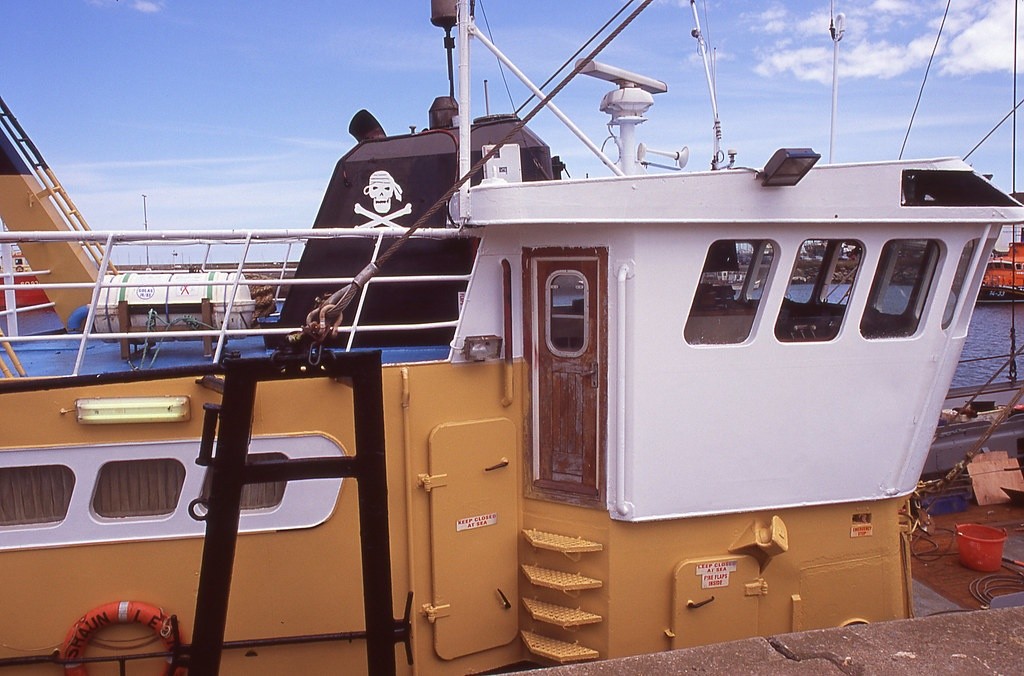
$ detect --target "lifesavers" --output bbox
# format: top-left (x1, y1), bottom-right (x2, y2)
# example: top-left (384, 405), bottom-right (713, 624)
top-left (62, 600), bottom-right (185, 676)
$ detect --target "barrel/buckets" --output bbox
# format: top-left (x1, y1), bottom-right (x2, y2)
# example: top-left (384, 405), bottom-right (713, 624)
top-left (954, 524), bottom-right (1007, 572)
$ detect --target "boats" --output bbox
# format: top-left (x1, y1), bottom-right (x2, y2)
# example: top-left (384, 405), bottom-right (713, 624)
top-left (0, 244), bottom-right (67, 337)
top-left (1, 0), bottom-right (1024, 675)
top-left (951, 163), bottom-right (1024, 307)
top-left (804, 240), bottom-right (851, 258)
